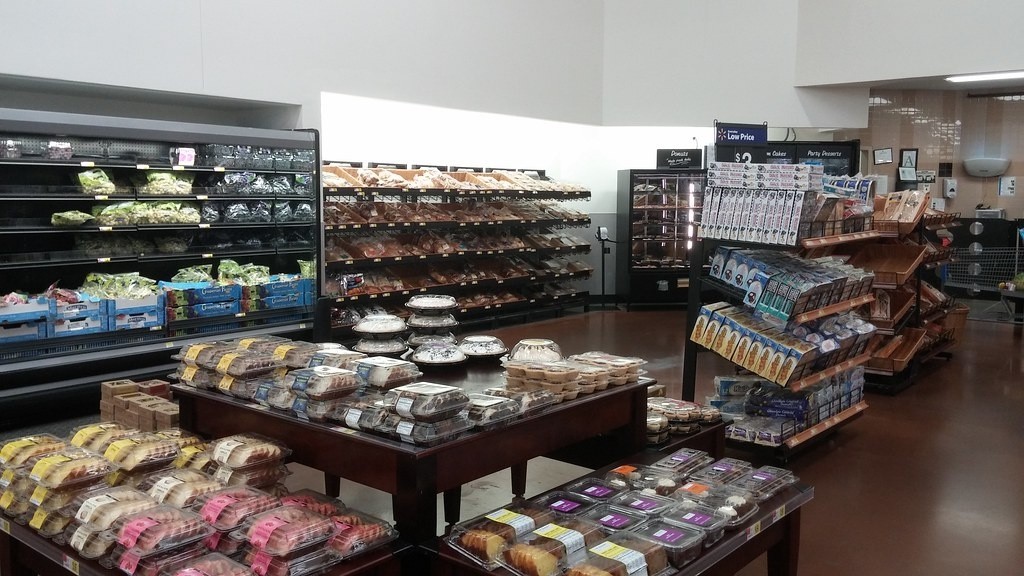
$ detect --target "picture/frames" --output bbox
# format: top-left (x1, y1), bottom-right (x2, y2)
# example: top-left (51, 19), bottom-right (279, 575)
top-left (873, 147), bottom-right (894, 165)
top-left (898, 166), bottom-right (918, 182)
top-left (899, 148), bottom-right (919, 171)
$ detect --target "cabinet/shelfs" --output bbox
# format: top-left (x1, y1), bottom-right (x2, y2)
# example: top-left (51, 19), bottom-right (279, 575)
top-left (0, 107), bottom-right (321, 436)
top-left (615, 168), bottom-right (728, 311)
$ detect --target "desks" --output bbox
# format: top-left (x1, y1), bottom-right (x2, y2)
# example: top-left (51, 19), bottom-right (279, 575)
top-left (420, 446), bottom-right (816, 576)
top-left (0, 509), bottom-right (413, 576)
top-left (646, 396), bottom-right (734, 462)
top-left (171, 352), bottom-right (657, 576)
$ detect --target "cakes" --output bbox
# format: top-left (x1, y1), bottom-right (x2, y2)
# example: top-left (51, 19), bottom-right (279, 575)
top-left (354, 295), bottom-right (561, 365)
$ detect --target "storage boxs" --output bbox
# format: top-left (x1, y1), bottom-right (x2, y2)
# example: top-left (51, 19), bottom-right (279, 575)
top-left (322, 165), bottom-right (592, 342)
top-left (689, 159), bottom-right (948, 447)
top-left (0, 273), bottom-right (317, 364)
top-left (101, 379), bottom-right (184, 433)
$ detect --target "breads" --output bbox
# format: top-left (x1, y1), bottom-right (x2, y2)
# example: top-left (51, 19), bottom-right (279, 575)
top-left (0, 421), bottom-right (389, 576)
top-left (457, 504), bottom-right (668, 576)
top-left (175, 335), bottom-right (554, 445)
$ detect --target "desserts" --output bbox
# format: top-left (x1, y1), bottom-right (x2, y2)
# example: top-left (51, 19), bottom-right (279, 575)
top-left (644, 397), bottom-right (720, 442)
top-left (503, 353), bottom-right (642, 400)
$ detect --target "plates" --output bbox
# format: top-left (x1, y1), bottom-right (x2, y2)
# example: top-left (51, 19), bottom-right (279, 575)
top-left (351, 302), bottom-right (509, 369)
